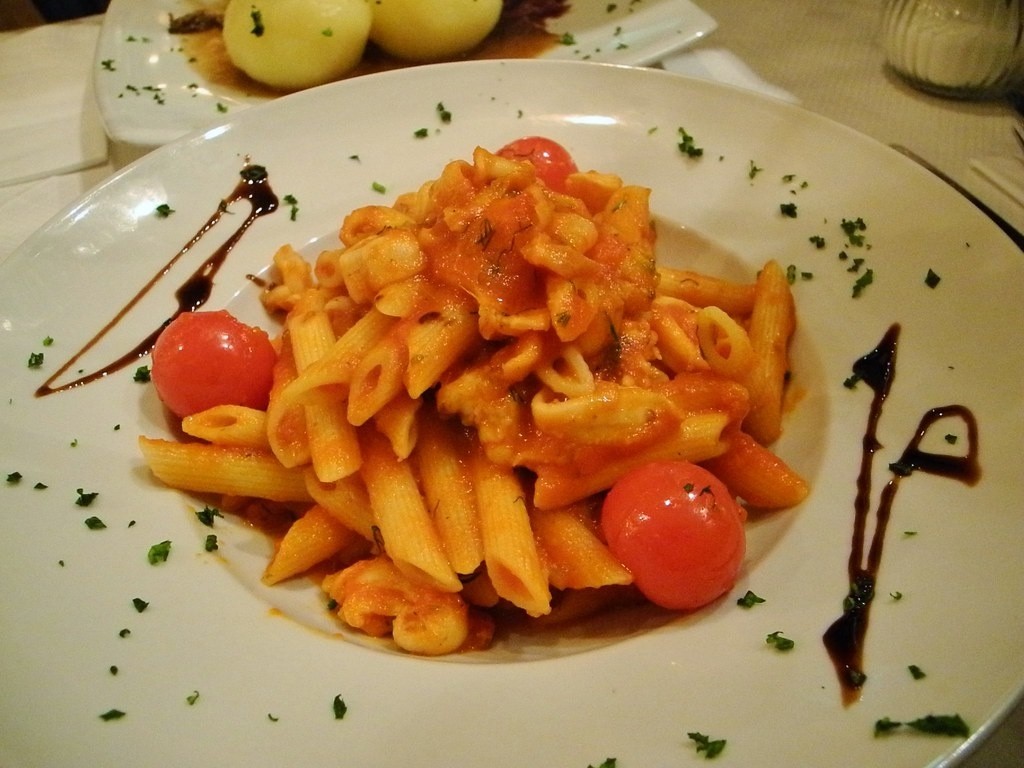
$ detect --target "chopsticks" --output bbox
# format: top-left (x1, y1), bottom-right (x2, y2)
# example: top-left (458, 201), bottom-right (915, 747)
top-left (968, 154), bottom-right (1024, 208)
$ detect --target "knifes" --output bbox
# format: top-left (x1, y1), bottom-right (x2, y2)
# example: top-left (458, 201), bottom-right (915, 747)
top-left (891, 144), bottom-right (1024, 251)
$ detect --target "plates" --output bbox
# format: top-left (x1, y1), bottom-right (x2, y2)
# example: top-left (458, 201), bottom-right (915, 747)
top-left (92, 0), bottom-right (719, 146)
top-left (0, 58), bottom-right (1024, 768)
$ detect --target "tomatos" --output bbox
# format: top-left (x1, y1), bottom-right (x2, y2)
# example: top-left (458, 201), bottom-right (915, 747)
top-left (147, 309), bottom-right (277, 418)
top-left (600, 460), bottom-right (745, 610)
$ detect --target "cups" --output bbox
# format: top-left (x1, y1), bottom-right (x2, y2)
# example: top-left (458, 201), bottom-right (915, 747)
top-left (879, 0), bottom-right (1024, 99)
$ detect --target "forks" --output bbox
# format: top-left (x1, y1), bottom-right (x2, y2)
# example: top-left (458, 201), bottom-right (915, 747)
top-left (1012, 119), bottom-right (1024, 153)
